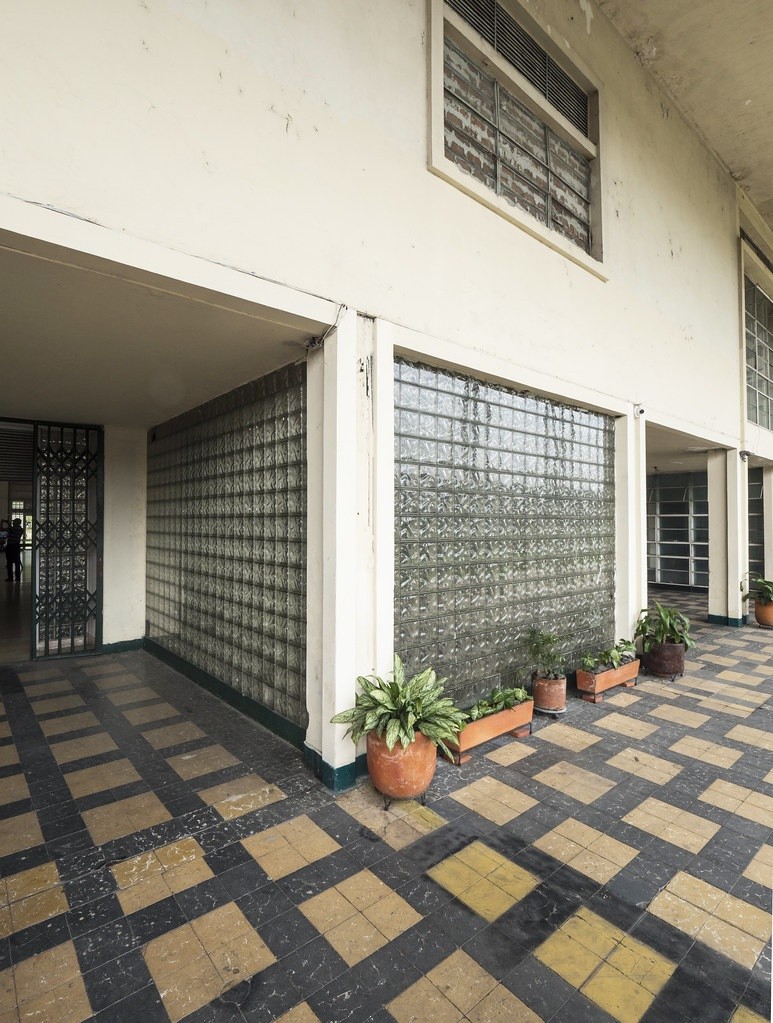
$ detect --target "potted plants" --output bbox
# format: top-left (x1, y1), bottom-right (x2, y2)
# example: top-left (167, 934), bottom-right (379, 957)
top-left (516, 624), bottom-right (574, 722)
top-left (634, 597), bottom-right (695, 683)
top-left (438, 686), bottom-right (537, 766)
top-left (739, 570), bottom-right (773, 628)
top-left (574, 638), bottom-right (639, 700)
top-left (330, 651), bottom-right (468, 812)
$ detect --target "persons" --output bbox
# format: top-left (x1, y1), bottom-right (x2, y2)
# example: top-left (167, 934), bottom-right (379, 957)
top-left (5, 518), bottom-right (23, 581)
